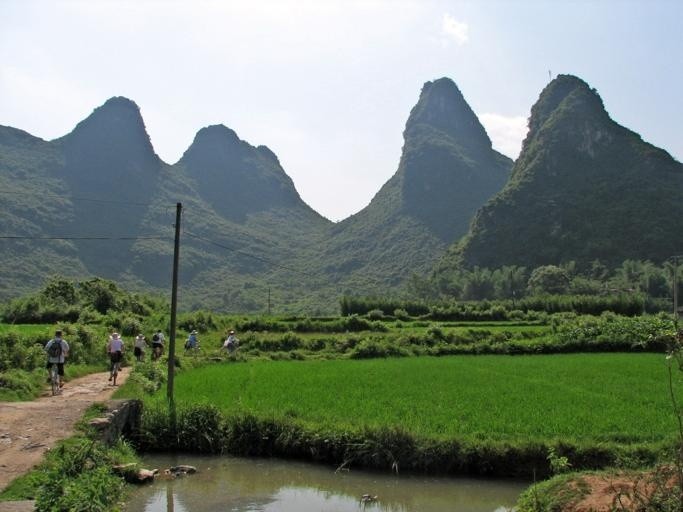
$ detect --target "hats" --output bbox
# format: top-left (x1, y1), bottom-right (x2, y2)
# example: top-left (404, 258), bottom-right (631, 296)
top-left (136, 334), bottom-right (144, 340)
top-left (190, 330), bottom-right (198, 335)
top-left (109, 333), bottom-right (121, 340)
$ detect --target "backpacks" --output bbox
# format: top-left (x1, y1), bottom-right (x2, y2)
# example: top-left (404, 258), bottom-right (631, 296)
top-left (47, 339), bottom-right (61, 361)
top-left (152, 334), bottom-right (159, 341)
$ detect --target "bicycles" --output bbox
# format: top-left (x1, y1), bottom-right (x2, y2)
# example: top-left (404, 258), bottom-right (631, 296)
top-left (135, 340), bottom-right (248, 363)
top-left (50, 364), bottom-right (63, 395)
top-left (112, 361), bottom-right (122, 385)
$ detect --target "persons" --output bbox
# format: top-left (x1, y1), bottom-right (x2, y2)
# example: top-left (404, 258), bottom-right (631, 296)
top-left (105, 332), bottom-right (127, 382)
top-left (222, 329), bottom-right (239, 354)
top-left (43, 329), bottom-right (70, 387)
top-left (132, 333), bottom-right (147, 361)
top-left (152, 328), bottom-right (167, 357)
top-left (183, 329), bottom-right (199, 352)
